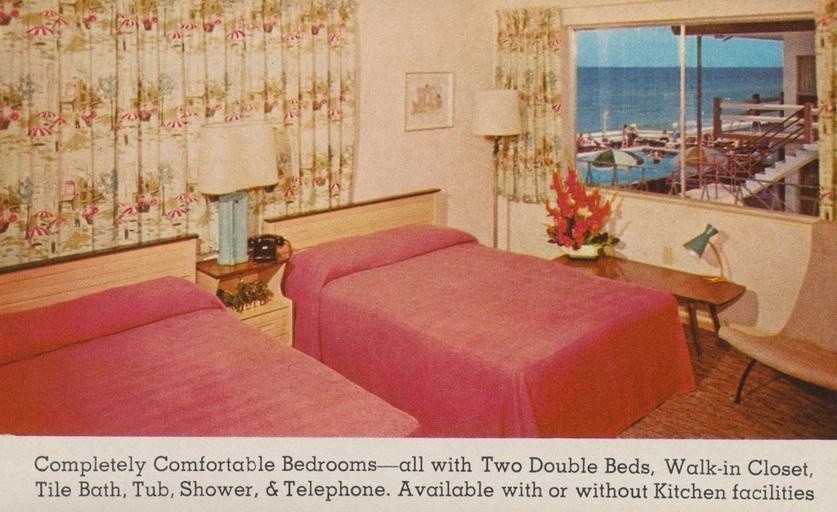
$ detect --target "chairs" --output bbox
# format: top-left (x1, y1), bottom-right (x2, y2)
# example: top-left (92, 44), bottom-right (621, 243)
top-left (718, 220), bottom-right (837, 405)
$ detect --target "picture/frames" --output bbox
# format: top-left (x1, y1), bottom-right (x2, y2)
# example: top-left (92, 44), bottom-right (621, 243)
top-left (405, 72), bottom-right (456, 132)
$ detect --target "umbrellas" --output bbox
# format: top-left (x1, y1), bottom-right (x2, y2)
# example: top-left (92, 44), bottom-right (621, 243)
top-left (591, 149), bottom-right (642, 182)
top-left (670, 145), bottom-right (724, 172)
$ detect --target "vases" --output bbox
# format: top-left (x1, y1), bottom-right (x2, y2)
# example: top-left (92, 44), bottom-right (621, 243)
top-left (562, 245), bottom-right (600, 259)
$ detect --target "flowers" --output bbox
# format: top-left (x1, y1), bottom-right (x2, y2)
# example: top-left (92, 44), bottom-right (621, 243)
top-left (546, 167), bottom-right (619, 258)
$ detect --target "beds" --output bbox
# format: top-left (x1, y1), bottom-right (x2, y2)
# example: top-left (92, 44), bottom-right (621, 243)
top-left (0, 233), bottom-right (422, 437)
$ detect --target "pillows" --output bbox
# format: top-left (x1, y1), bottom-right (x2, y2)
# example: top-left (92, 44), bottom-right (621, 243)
top-left (0, 276), bottom-right (226, 366)
top-left (289, 223), bottom-right (479, 301)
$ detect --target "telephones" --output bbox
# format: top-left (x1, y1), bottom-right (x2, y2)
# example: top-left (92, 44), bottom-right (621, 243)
top-left (247, 234), bottom-right (284, 263)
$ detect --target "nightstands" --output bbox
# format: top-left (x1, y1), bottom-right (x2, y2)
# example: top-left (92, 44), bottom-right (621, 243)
top-left (197, 250), bottom-right (294, 348)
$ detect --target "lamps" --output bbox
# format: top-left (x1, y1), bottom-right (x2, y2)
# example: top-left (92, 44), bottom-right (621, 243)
top-left (470, 90), bottom-right (522, 249)
top-left (197, 120), bottom-right (278, 267)
top-left (684, 224), bottom-right (728, 284)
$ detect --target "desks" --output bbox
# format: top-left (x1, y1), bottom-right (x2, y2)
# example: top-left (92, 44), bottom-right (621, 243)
top-left (552, 255), bottom-right (745, 357)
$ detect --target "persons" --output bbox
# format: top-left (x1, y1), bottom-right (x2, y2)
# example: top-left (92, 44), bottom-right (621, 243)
top-left (629, 122), bottom-right (639, 146)
top-left (619, 124), bottom-right (633, 149)
top-left (647, 148), bottom-right (655, 158)
top-left (650, 150), bottom-right (661, 165)
top-left (587, 132), bottom-right (593, 140)
top-left (577, 133), bottom-right (584, 144)
